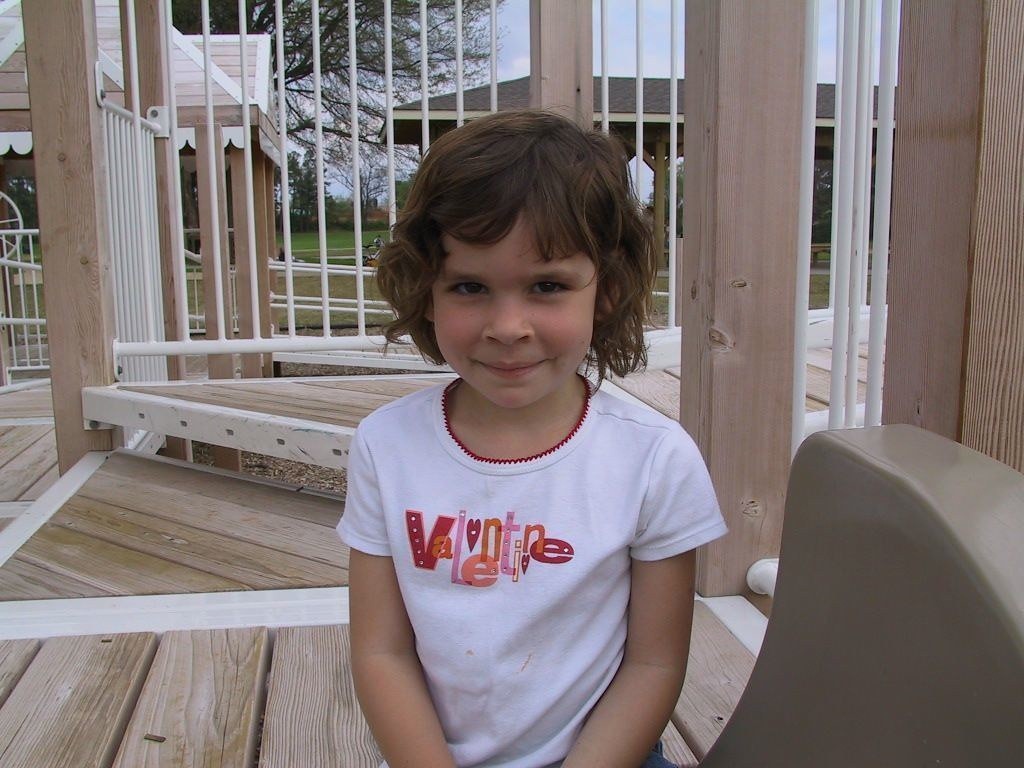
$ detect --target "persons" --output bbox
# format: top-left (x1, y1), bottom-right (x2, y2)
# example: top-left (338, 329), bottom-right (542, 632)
top-left (332, 108), bottom-right (728, 768)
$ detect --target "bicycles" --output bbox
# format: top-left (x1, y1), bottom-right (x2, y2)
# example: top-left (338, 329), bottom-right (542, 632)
top-left (344, 234), bottom-right (387, 268)
